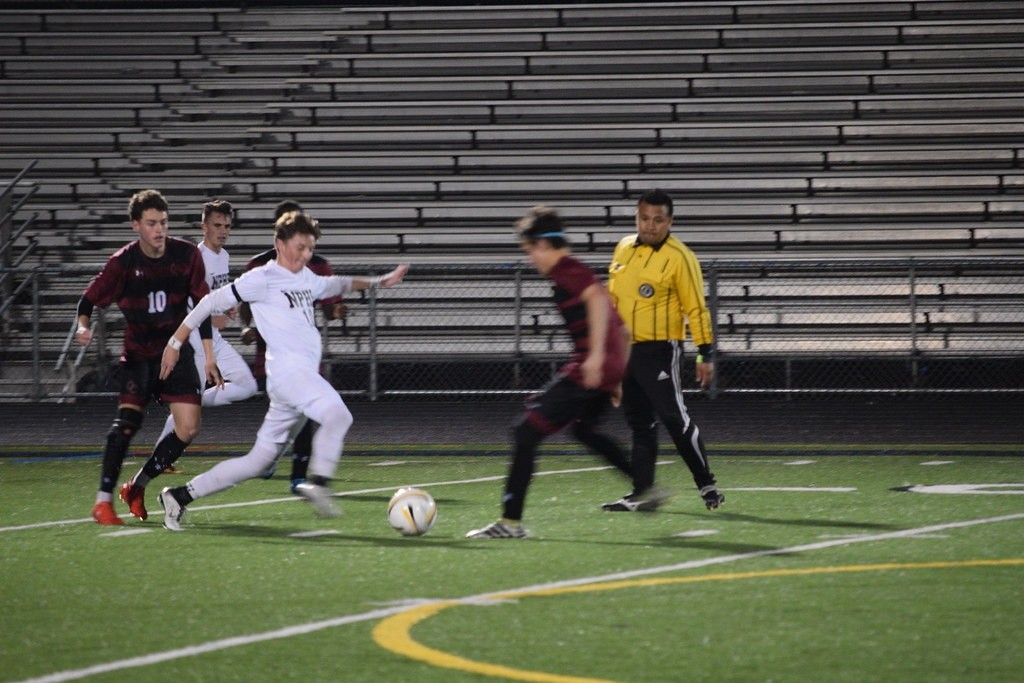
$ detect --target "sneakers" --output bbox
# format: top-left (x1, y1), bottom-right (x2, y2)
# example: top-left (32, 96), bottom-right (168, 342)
top-left (700, 485), bottom-right (724, 510)
top-left (602, 493), bottom-right (656, 512)
top-left (94, 499), bottom-right (123, 525)
top-left (296, 482), bottom-right (339, 517)
top-left (466, 520), bottom-right (527, 540)
top-left (119, 475), bottom-right (148, 520)
top-left (158, 485), bottom-right (188, 532)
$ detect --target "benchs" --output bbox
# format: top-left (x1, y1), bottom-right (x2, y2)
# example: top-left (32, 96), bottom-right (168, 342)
top-left (1, 0), bottom-right (1024, 381)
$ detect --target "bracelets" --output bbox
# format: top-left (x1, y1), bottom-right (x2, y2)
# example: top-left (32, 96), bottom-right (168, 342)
top-left (369, 278), bottom-right (378, 289)
top-left (168, 337), bottom-right (181, 350)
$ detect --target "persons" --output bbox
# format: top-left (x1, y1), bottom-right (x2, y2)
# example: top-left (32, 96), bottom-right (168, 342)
top-left (239, 199), bottom-right (349, 495)
top-left (76, 190), bottom-right (225, 526)
top-left (466, 206), bottom-right (668, 540)
top-left (600, 189), bottom-right (725, 512)
top-left (152, 201), bottom-right (258, 474)
top-left (156, 211), bottom-right (409, 532)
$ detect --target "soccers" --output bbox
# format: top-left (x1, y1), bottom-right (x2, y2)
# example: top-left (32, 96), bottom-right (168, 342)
top-left (388, 488), bottom-right (437, 536)
